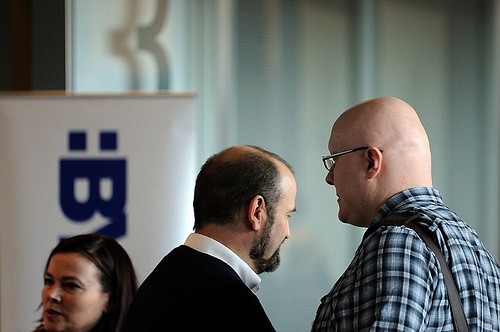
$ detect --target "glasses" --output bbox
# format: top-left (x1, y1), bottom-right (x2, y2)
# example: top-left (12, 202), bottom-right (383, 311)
top-left (316, 144), bottom-right (383, 172)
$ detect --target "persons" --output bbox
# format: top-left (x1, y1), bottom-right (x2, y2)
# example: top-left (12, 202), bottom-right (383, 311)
top-left (31, 234), bottom-right (137, 332)
top-left (120, 145), bottom-right (298, 332)
top-left (309, 96), bottom-right (498, 332)
